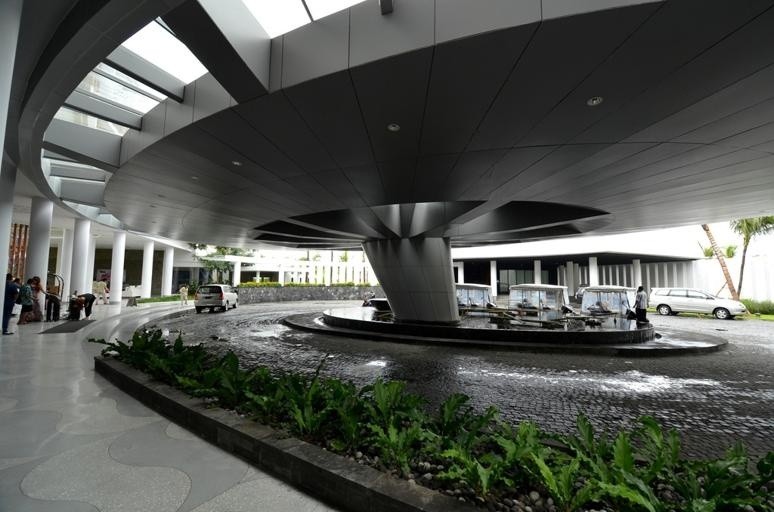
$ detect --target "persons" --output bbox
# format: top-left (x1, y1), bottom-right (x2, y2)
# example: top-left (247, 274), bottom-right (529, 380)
top-left (180, 284), bottom-right (190, 307)
top-left (633, 286), bottom-right (647, 322)
top-left (76, 294), bottom-right (96, 320)
top-left (2, 274), bottom-right (50, 334)
top-left (96, 279), bottom-right (112, 305)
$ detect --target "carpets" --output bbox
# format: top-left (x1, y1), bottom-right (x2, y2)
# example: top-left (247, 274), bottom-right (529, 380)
top-left (37, 318), bottom-right (97, 333)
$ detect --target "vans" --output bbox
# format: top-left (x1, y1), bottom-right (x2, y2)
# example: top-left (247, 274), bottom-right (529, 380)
top-left (647, 286), bottom-right (747, 320)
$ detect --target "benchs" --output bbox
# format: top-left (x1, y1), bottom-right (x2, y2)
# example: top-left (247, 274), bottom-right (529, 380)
top-left (95, 295), bottom-right (141, 307)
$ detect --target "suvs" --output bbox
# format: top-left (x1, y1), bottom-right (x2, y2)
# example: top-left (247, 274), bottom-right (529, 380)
top-left (193, 283), bottom-right (239, 313)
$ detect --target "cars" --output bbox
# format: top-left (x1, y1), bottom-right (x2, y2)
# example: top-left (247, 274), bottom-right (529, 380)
top-left (575, 284), bottom-right (590, 304)
